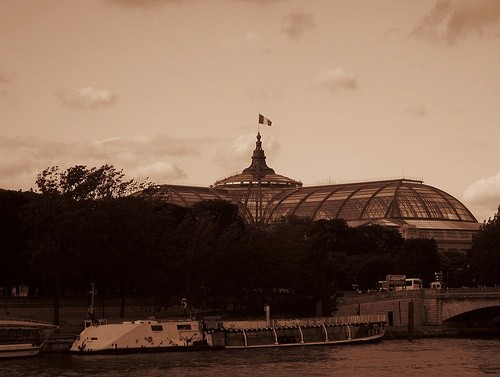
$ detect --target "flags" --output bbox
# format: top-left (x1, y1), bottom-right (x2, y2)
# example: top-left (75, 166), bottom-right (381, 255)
top-left (259, 114), bottom-right (272, 126)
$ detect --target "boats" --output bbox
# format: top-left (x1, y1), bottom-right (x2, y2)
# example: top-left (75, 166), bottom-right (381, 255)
top-left (69, 283), bottom-right (204, 354)
top-left (0, 316), bottom-right (59, 358)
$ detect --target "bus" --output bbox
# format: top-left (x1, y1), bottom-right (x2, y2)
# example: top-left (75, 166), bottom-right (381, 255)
top-left (378, 278), bottom-right (422, 293)
